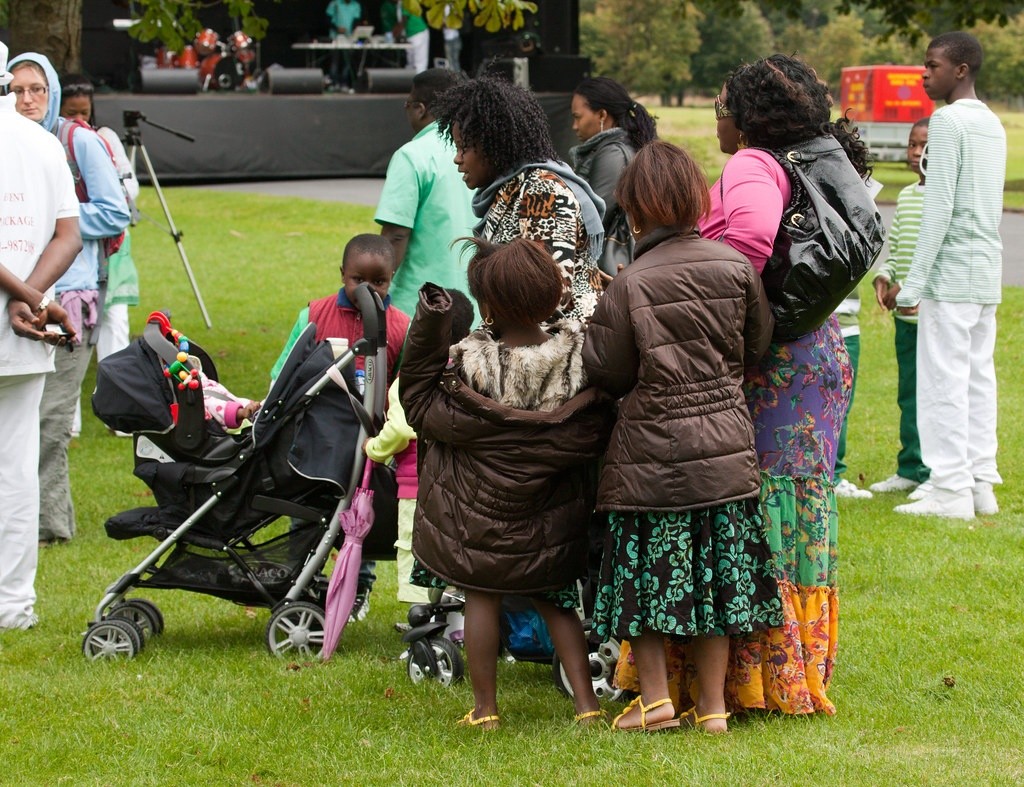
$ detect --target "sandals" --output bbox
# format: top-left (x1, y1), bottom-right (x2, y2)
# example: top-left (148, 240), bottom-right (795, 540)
top-left (611, 695), bottom-right (680, 734)
top-left (457, 708), bottom-right (501, 730)
top-left (575, 707), bottom-right (607, 727)
top-left (676, 704), bottom-right (730, 732)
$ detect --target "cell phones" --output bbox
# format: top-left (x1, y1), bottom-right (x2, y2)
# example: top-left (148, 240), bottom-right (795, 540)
top-left (44, 324), bottom-right (77, 340)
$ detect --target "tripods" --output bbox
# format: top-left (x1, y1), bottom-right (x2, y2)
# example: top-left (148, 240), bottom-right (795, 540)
top-left (117, 107), bottom-right (213, 330)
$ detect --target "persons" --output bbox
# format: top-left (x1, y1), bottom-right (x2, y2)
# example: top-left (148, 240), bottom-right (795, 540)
top-left (0, 52), bottom-right (871, 733)
top-left (894, 32), bottom-right (1007, 520)
top-left (869, 117), bottom-right (930, 499)
top-left (378, 0), bottom-right (430, 72)
top-left (833, 289), bottom-right (873, 502)
top-left (442, 4), bottom-right (464, 73)
top-left (325, 0), bottom-right (362, 92)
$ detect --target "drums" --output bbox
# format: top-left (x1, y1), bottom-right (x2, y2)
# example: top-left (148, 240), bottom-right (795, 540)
top-left (156, 28), bottom-right (255, 92)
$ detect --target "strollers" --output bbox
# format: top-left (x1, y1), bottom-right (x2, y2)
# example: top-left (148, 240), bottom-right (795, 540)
top-left (396, 571), bottom-right (632, 704)
top-left (82, 281), bottom-right (388, 670)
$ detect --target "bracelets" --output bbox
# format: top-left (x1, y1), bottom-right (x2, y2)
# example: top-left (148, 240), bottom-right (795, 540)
top-left (32, 295), bottom-right (50, 314)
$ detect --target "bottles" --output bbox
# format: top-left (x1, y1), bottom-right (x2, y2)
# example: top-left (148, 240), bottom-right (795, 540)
top-left (355, 369), bottom-right (364, 395)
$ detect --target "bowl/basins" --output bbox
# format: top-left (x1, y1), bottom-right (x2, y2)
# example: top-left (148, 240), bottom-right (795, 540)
top-left (228, 559), bottom-right (293, 592)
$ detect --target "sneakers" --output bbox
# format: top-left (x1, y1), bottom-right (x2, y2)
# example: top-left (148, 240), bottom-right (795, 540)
top-left (832, 479), bottom-right (872, 499)
top-left (893, 496), bottom-right (975, 521)
top-left (907, 478), bottom-right (936, 500)
top-left (971, 480), bottom-right (998, 515)
top-left (871, 474), bottom-right (919, 493)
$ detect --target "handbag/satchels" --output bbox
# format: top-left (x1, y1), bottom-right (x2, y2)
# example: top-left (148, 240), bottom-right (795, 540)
top-left (721, 132), bottom-right (885, 344)
top-left (592, 140), bottom-right (637, 277)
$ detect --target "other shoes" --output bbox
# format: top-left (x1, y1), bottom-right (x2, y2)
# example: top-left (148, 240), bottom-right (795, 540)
top-left (38, 538), bottom-right (50, 548)
top-left (349, 587), bottom-right (371, 622)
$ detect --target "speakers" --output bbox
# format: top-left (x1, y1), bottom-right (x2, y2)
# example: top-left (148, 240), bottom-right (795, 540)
top-left (258, 67), bottom-right (323, 95)
top-left (79, 0), bottom-right (135, 92)
top-left (354, 68), bottom-right (417, 93)
top-left (131, 68), bottom-right (200, 95)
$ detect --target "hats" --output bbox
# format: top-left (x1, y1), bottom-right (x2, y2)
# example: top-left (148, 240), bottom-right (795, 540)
top-left (0, 41), bottom-right (14, 85)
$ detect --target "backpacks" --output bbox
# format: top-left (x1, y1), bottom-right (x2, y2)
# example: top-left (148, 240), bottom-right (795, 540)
top-left (60, 118), bottom-right (124, 257)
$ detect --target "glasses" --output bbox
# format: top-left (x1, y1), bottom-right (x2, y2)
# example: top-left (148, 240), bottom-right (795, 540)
top-left (714, 95), bottom-right (734, 118)
top-left (10, 86), bottom-right (47, 96)
top-left (62, 83), bottom-right (95, 95)
